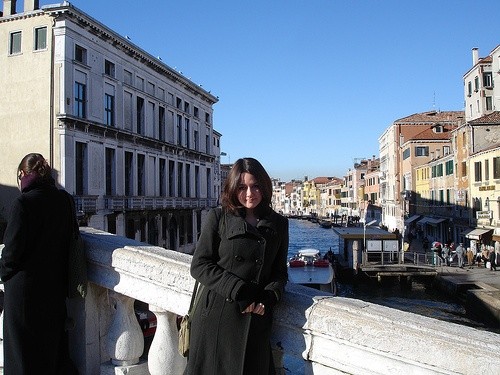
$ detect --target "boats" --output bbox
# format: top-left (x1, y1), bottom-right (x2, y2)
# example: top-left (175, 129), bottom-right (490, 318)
top-left (287, 247), bottom-right (337, 295)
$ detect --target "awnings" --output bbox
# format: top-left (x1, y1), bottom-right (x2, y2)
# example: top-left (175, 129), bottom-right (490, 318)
top-left (405, 214), bottom-right (446, 225)
top-left (466, 229), bottom-right (493, 240)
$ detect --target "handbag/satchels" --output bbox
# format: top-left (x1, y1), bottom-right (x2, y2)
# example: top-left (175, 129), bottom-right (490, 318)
top-left (178, 316), bottom-right (191, 357)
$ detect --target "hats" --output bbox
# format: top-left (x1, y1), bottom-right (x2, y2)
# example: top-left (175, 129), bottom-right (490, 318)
top-left (459, 243), bottom-right (464, 245)
top-left (445, 244), bottom-right (448, 246)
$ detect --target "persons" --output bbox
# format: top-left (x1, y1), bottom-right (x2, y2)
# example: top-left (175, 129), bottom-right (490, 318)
top-left (0, 152), bottom-right (83, 375)
top-left (330, 213), bottom-right (361, 224)
top-left (380, 225), bottom-right (496, 271)
top-left (183, 158), bottom-right (289, 375)
